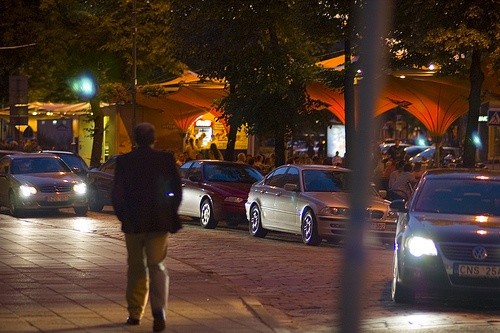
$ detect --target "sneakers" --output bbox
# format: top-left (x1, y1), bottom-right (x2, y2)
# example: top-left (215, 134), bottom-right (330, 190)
top-left (152, 302), bottom-right (166, 333)
top-left (127, 319), bottom-right (140, 325)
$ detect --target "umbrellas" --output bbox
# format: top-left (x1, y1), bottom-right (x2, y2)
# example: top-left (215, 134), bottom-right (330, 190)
top-left (0, 99), bottom-right (128, 119)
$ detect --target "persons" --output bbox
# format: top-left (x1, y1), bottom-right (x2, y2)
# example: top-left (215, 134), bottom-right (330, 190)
top-left (0, 137), bottom-right (61, 153)
top-left (112, 122), bottom-right (183, 333)
top-left (380, 151), bottom-right (463, 177)
top-left (388, 137), bottom-right (404, 171)
top-left (175, 132), bottom-right (218, 167)
top-left (388, 162), bottom-right (419, 201)
top-left (235, 147), bottom-right (346, 177)
top-left (209, 143), bottom-right (224, 160)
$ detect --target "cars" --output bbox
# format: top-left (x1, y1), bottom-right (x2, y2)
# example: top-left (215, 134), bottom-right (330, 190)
top-left (380, 138), bottom-right (463, 169)
top-left (287, 133), bottom-right (326, 158)
top-left (34, 149), bottom-right (89, 179)
top-left (245, 163), bottom-right (400, 248)
top-left (0, 152), bottom-right (90, 218)
top-left (87, 154), bottom-right (120, 211)
top-left (389, 167), bottom-right (500, 311)
top-left (177, 159), bottom-right (264, 229)
